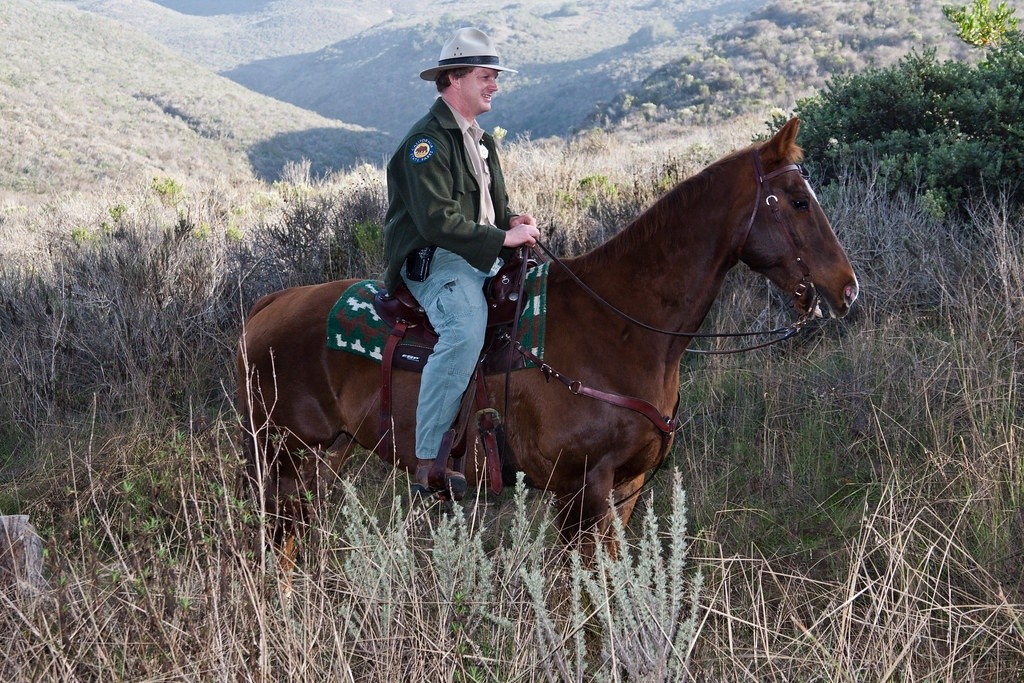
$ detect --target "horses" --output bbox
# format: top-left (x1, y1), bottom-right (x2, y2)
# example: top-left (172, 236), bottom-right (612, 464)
top-left (231, 115), bottom-right (861, 638)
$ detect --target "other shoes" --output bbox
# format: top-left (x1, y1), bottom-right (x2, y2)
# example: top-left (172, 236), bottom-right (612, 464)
top-left (412, 459), bottom-right (467, 496)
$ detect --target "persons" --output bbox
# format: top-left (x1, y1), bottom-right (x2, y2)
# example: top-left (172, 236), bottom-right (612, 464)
top-left (380, 28), bottom-right (540, 498)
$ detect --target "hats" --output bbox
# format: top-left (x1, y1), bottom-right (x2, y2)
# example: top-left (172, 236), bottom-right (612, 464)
top-left (420, 27), bottom-right (518, 81)
